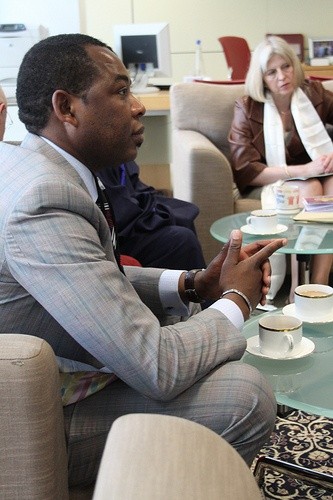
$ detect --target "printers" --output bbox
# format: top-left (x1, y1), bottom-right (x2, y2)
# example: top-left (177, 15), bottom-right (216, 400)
top-left (0, 20), bottom-right (37, 103)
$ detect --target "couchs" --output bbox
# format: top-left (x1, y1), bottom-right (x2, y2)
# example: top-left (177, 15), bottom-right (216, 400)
top-left (0, 84), bottom-right (333, 499)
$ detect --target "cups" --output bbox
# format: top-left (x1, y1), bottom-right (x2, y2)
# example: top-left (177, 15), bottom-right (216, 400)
top-left (246, 210), bottom-right (278, 233)
top-left (273, 185), bottom-right (301, 214)
top-left (257, 315), bottom-right (304, 356)
top-left (294, 283), bottom-right (333, 321)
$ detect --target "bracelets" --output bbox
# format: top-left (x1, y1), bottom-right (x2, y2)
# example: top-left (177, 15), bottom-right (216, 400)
top-left (219, 289), bottom-right (252, 317)
top-left (184, 268), bottom-right (206, 303)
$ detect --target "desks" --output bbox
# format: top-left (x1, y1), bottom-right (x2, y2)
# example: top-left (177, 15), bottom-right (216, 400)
top-left (231, 303), bottom-right (332, 481)
top-left (129, 65), bottom-right (333, 110)
top-left (209, 207), bottom-right (333, 285)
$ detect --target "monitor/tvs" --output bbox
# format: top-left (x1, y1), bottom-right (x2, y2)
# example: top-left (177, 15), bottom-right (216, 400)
top-left (112, 23), bottom-right (173, 94)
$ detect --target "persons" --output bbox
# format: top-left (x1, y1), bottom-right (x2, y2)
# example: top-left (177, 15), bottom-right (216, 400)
top-left (0, 34), bottom-right (288, 488)
top-left (227, 34), bottom-right (333, 286)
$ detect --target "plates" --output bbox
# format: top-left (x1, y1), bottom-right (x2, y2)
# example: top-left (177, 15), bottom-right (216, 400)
top-left (243, 334), bottom-right (315, 361)
top-left (240, 224), bottom-right (288, 236)
top-left (281, 302), bottom-right (333, 324)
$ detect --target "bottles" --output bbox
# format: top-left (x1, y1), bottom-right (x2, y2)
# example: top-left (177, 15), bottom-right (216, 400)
top-left (192, 40), bottom-right (207, 78)
